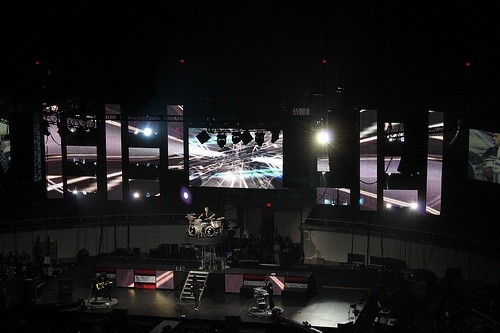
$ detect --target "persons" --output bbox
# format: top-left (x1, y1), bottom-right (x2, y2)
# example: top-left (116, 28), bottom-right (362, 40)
top-left (227, 231), bottom-right (295, 268)
top-left (261, 278), bottom-right (274, 310)
top-left (93, 272), bottom-right (112, 303)
top-left (0, 235), bottom-right (50, 308)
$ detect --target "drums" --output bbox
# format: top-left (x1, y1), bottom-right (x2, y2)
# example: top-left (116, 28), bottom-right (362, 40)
top-left (210, 222), bottom-right (220, 229)
top-left (188, 225), bottom-right (202, 236)
top-left (205, 226), bottom-right (216, 237)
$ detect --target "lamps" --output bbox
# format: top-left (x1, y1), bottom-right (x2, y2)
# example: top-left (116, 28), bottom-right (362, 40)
top-left (197, 124), bottom-right (283, 151)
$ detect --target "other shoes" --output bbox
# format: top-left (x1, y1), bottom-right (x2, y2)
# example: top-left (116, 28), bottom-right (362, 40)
top-left (194, 307), bottom-right (199, 311)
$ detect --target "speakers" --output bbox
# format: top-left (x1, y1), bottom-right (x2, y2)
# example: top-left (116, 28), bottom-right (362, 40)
top-left (58, 279), bottom-right (74, 295)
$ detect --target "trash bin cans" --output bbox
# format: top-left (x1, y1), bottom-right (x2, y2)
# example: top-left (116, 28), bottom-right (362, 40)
top-left (58, 278), bottom-right (74, 297)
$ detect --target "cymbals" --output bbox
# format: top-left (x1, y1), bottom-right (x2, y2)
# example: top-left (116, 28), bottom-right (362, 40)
top-left (217, 217), bottom-right (225, 220)
